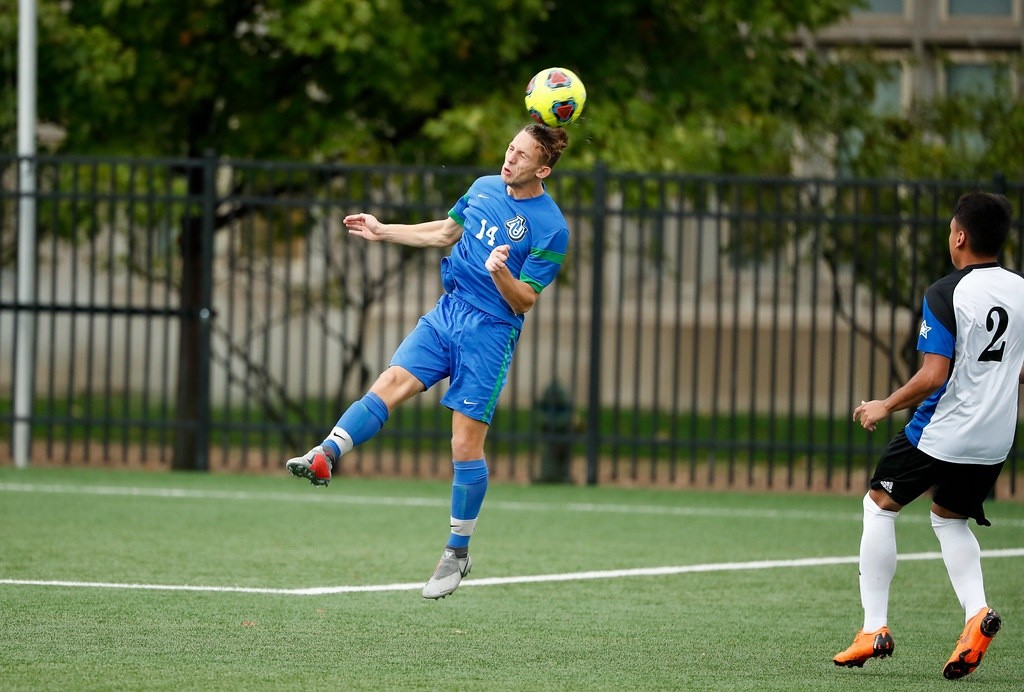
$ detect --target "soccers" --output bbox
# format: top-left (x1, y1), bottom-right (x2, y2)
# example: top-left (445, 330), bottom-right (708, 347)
top-left (525, 67), bottom-right (587, 128)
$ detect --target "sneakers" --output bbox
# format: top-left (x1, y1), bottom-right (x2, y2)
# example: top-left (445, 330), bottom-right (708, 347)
top-left (422, 549), bottom-right (472, 600)
top-left (286, 444), bottom-right (333, 487)
top-left (833, 626), bottom-right (894, 669)
top-left (942, 607), bottom-right (1002, 680)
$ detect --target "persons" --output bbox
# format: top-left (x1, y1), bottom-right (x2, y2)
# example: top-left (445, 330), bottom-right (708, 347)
top-left (832, 188), bottom-right (1024, 681)
top-left (285, 123), bottom-right (572, 599)
